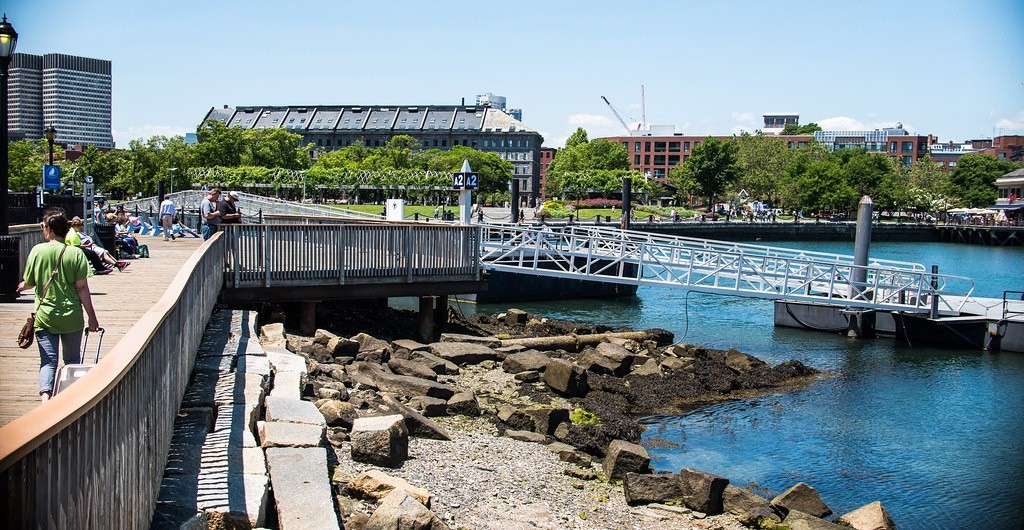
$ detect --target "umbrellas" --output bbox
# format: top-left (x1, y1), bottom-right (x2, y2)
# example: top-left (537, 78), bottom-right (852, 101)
top-left (947, 207), bottom-right (999, 225)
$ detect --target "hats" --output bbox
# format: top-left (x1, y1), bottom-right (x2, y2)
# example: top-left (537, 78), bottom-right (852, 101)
top-left (230, 191), bottom-right (239, 201)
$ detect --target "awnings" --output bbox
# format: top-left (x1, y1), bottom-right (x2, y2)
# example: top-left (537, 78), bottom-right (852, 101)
top-left (986, 205), bottom-right (1024, 210)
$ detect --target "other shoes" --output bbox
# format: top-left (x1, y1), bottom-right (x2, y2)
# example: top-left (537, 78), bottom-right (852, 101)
top-left (163, 238), bottom-right (169, 241)
top-left (94, 267), bottom-right (113, 275)
top-left (238, 264), bottom-right (245, 271)
top-left (226, 264), bottom-right (232, 272)
top-left (171, 234), bottom-right (175, 240)
top-left (115, 261), bottom-right (131, 271)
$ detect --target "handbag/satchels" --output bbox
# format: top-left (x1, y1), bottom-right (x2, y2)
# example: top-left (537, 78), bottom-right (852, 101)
top-left (138, 245), bottom-right (149, 258)
top-left (18, 317), bottom-right (35, 349)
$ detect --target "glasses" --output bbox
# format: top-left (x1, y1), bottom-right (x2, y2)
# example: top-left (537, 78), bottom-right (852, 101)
top-left (40, 223), bottom-right (48, 229)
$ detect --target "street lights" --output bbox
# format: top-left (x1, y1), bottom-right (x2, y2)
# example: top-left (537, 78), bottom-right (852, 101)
top-left (44, 122), bottom-right (57, 197)
top-left (442, 187), bottom-right (447, 220)
top-left (0, 11), bottom-right (18, 236)
top-left (165, 167), bottom-right (178, 194)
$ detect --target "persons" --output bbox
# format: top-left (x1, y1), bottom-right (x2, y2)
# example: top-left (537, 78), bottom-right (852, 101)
top-left (478, 210), bottom-right (483, 222)
top-left (159, 195), bottom-right (176, 241)
top-left (671, 209), bottom-right (678, 221)
top-left (220, 191), bottom-right (241, 248)
top-left (536, 197), bottom-right (539, 207)
top-left (521, 210), bottom-right (523, 219)
top-left (435, 207), bottom-right (439, 219)
top-left (172, 210), bottom-right (196, 232)
top-left (201, 187), bottom-right (220, 241)
top-left (16, 207), bottom-right (99, 404)
top-left (64, 198), bottom-right (152, 274)
top-left (730, 209), bottom-right (776, 221)
top-left (201, 182), bottom-right (210, 191)
top-left (876, 213), bottom-right (881, 223)
top-left (816, 214), bottom-right (819, 222)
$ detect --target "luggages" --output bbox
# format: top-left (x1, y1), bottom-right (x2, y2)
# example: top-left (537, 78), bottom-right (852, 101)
top-left (53, 326), bottom-right (105, 399)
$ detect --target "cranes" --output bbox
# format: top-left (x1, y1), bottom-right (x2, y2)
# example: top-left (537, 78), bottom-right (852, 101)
top-left (600, 84), bottom-right (646, 136)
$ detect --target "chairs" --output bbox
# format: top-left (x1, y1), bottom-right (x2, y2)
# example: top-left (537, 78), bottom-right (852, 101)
top-left (124, 217), bottom-right (201, 240)
